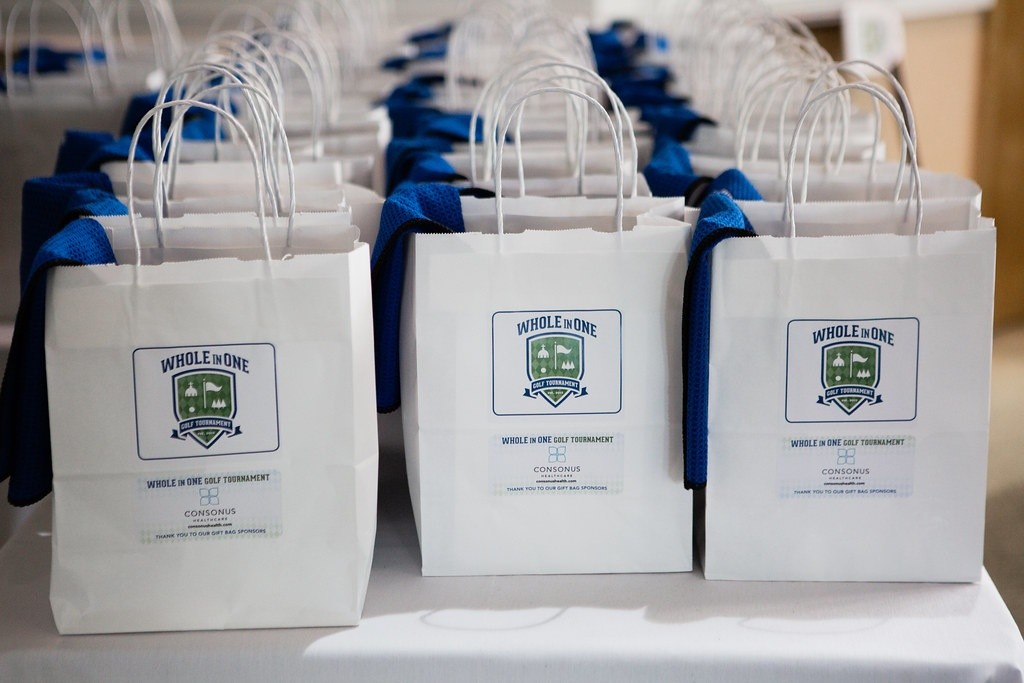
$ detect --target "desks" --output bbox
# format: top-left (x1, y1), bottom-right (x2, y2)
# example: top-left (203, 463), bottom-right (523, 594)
top-left (0, 402), bottom-right (1024, 683)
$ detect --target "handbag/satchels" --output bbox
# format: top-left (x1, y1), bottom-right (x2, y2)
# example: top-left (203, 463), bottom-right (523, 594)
top-left (0, 0), bottom-right (1003, 632)
